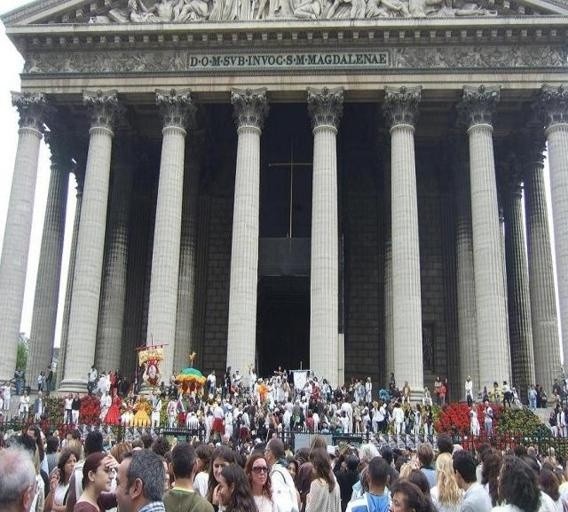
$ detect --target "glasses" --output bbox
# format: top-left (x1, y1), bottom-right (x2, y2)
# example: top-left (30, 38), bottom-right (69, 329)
top-left (251, 466), bottom-right (270, 473)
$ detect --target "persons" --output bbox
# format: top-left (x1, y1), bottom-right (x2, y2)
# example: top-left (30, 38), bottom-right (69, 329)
top-left (126, 0), bottom-right (499, 21)
top-left (1, 360), bottom-right (567, 512)
